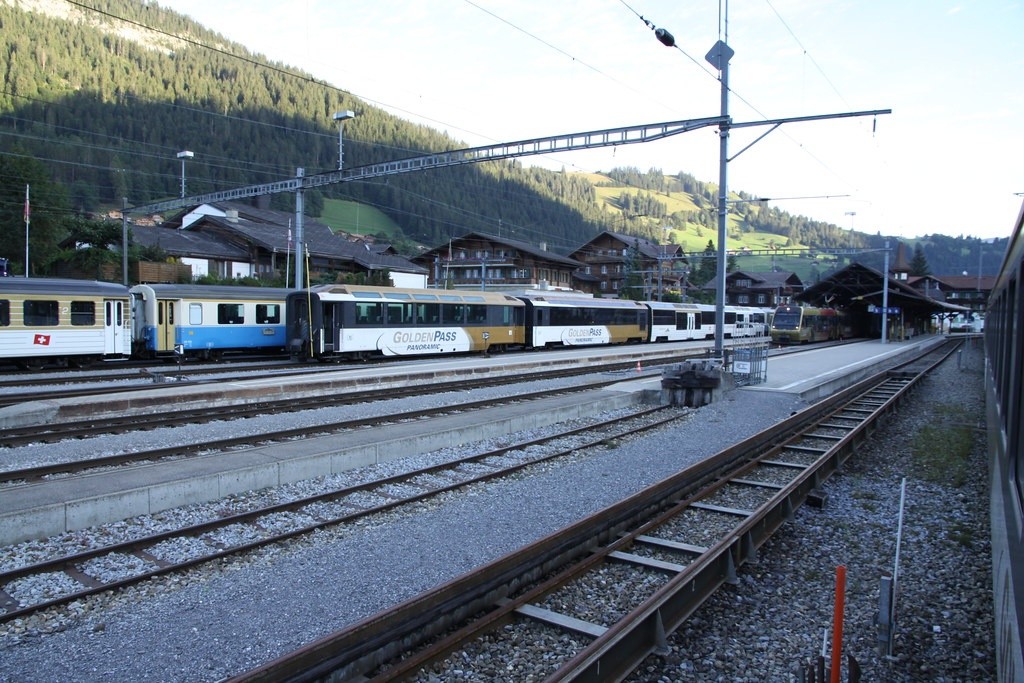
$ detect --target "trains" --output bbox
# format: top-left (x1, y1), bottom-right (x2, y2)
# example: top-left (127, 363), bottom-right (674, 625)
top-left (769, 304), bottom-right (881, 346)
top-left (981, 197), bottom-right (1024, 683)
top-left (0, 272), bottom-right (775, 372)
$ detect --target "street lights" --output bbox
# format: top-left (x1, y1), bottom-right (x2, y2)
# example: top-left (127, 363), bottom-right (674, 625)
top-left (444, 236), bottom-right (463, 289)
top-left (332, 110), bottom-right (355, 180)
top-left (661, 225), bottom-right (674, 259)
top-left (176, 151), bottom-right (195, 205)
